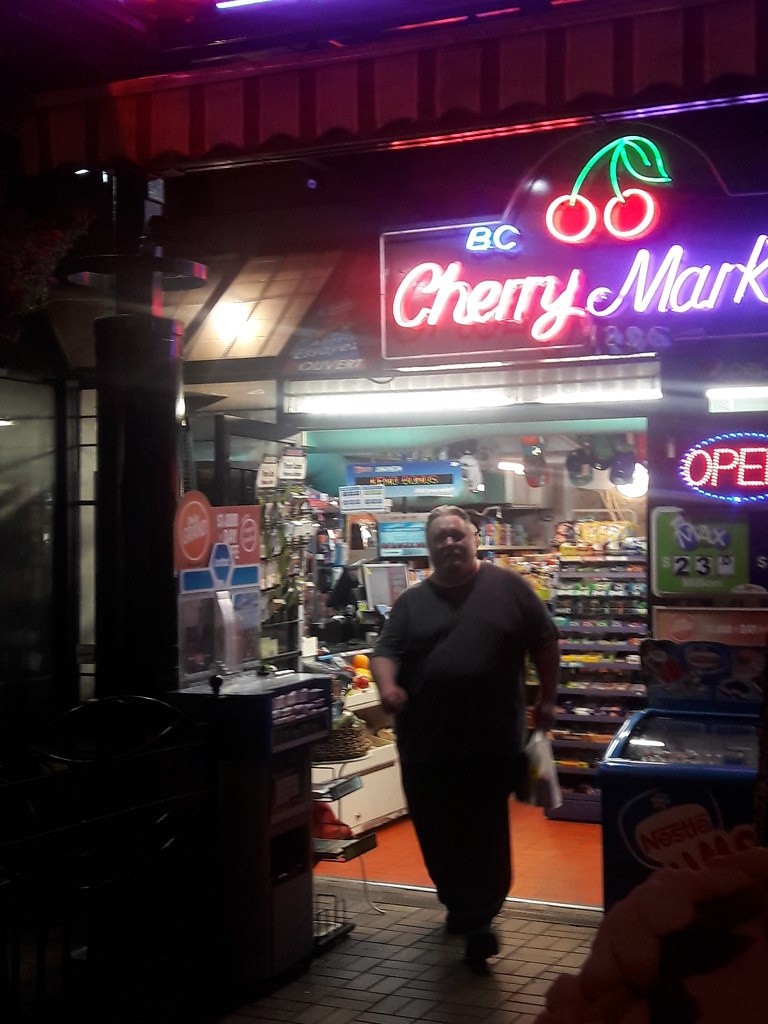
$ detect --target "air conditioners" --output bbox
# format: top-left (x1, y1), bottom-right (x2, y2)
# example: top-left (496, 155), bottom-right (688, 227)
top-left (607, 435), bottom-right (636, 486)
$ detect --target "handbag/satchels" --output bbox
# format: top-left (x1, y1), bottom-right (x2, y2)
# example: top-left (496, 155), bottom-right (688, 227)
top-left (510, 726), bottom-right (563, 811)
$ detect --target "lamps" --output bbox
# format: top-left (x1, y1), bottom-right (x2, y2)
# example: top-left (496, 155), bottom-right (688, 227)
top-left (457, 438), bottom-right (484, 492)
top-left (564, 448), bottom-right (593, 487)
top-left (519, 435), bottom-right (548, 487)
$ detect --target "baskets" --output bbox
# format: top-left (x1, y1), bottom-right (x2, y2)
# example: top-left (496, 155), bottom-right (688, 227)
top-left (302, 717), bottom-right (369, 761)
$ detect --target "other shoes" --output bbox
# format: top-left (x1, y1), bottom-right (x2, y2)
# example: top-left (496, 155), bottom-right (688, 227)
top-left (445, 907), bottom-right (463, 939)
top-left (459, 926), bottom-right (501, 963)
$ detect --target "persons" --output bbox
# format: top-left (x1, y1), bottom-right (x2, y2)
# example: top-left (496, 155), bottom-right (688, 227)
top-left (368, 505), bottom-right (560, 969)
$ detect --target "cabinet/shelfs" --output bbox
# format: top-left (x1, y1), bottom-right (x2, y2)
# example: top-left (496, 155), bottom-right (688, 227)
top-left (252, 483), bottom-right (647, 841)
